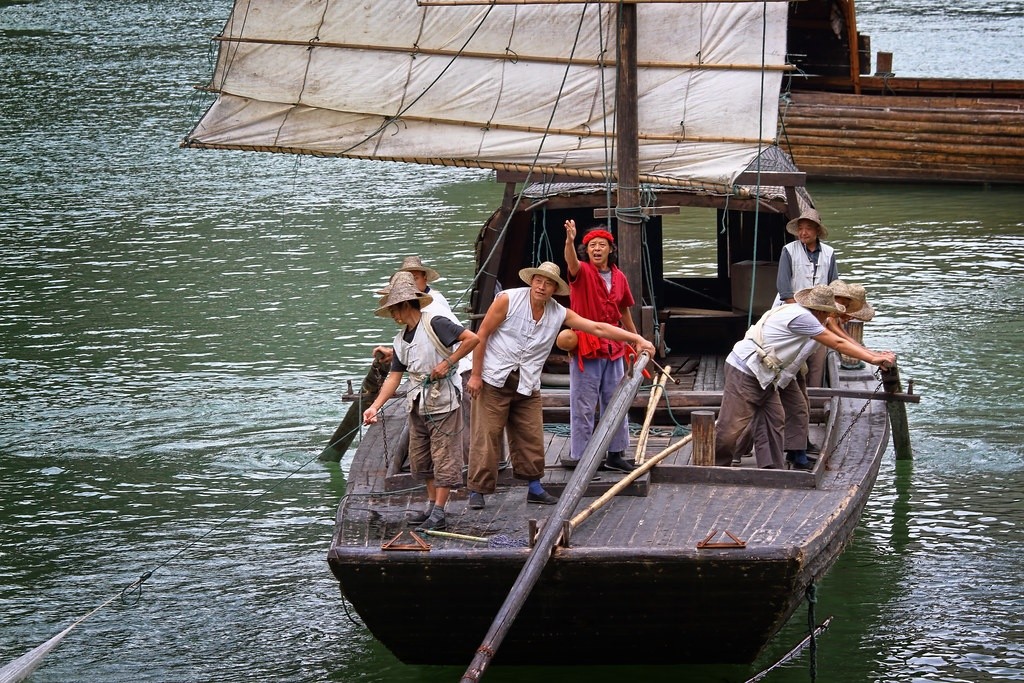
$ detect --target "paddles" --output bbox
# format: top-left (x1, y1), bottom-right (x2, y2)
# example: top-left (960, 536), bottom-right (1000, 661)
top-left (316, 350), bottom-right (394, 463)
top-left (456, 348), bottom-right (654, 683)
top-left (877, 354), bottom-right (920, 463)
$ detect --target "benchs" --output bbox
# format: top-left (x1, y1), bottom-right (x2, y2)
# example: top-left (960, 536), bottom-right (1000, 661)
top-left (658, 305), bottom-right (740, 323)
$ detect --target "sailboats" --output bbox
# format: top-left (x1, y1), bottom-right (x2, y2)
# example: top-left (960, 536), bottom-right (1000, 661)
top-left (172, 0), bottom-right (921, 673)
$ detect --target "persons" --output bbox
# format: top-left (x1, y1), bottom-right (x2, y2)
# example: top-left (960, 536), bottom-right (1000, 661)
top-left (770, 207), bottom-right (840, 393)
top-left (467, 262), bottom-right (655, 508)
top-left (363, 256), bottom-right (477, 531)
top-left (564, 218), bottom-right (640, 473)
top-left (713, 279), bottom-right (896, 472)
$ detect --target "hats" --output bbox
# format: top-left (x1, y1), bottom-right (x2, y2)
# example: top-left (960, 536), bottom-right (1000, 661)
top-left (398, 256), bottom-right (441, 282)
top-left (793, 286), bottom-right (847, 314)
top-left (786, 209), bottom-right (829, 240)
top-left (844, 283), bottom-right (875, 321)
top-left (374, 283), bottom-right (433, 318)
top-left (828, 279), bottom-right (864, 313)
top-left (378, 270), bottom-right (406, 295)
top-left (519, 262), bottom-right (571, 296)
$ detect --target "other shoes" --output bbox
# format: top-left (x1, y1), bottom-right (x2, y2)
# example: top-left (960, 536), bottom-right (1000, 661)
top-left (732, 456), bottom-right (743, 463)
top-left (744, 451), bottom-right (752, 456)
top-left (806, 443), bottom-right (821, 455)
top-left (416, 515), bottom-right (446, 532)
top-left (468, 492), bottom-right (485, 507)
top-left (408, 511), bottom-right (430, 524)
top-left (527, 491), bottom-right (558, 504)
top-left (603, 457), bottom-right (635, 473)
top-left (786, 451), bottom-right (815, 471)
